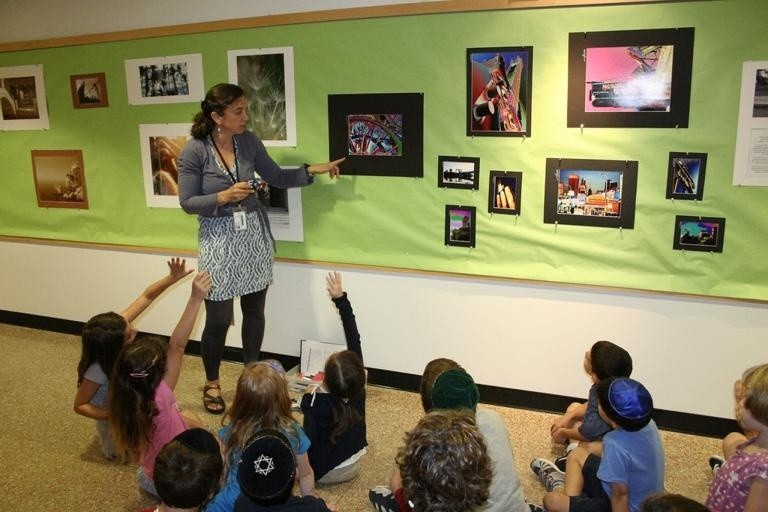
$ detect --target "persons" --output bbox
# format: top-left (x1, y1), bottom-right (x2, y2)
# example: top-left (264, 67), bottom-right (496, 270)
top-left (639, 494), bottom-right (716, 512)
top-left (704, 363), bottom-right (767, 511)
top-left (708, 380), bottom-right (758, 484)
top-left (552, 341), bottom-right (632, 470)
top-left (231, 431), bottom-right (330, 511)
top-left (392, 408), bottom-right (496, 511)
top-left (102, 272), bottom-right (215, 501)
top-left (369, 358), bottom-right (547, 512)
top-left (177, 82), bottom-right (347, 414)
top-left (529, 376), bottom-right (666, 512)
top-left (208, 358), bottom-right (339, 510)
top-left (74, 256), bottom-right (195, 461)
top-left (129, 428), bottom-right (227, 511)
top-left (300, 271), bottom-right (369, 484)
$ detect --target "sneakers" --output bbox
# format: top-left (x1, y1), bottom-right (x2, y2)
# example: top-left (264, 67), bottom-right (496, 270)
top-left (530, 459), bottom-right (566, 490)
top-left (555, 449), bottom-right (571, 473)
top-left (369, 487), bottom-right (399, 512)
top-left (708, 455), bottom-right (726, 472)
top-left (527, 502), bottom-right (546, 512)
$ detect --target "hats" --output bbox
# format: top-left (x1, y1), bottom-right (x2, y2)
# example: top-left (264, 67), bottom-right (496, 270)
top-left (599, 378), bottom-right (653, 432)
top-left (590, 341), bottom-right (632, 381)
top-left (421, 357), bottom-right (478, 412)
top-left (237, 429), bottom-right (296, 500)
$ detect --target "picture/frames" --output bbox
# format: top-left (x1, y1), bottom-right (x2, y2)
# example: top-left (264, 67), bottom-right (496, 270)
top-left (544, 157), bottom-right (638, 230)
top-left (567, 26), bottom-right (695, 128)
top-left (438, 155), bottom-right (480, 191)
top-left (466, 46), bottom-right (533, 137)
top-left (31, 149), bottom-right (89, 209)
top-left (70, 72), bottom-right (109, 110)
top-left (226, 47), bottom-right (297, 149)
top-left (0, 63), bottom-right (50, 131)
top-left (136, 123), bottom-right (202, 209)
top-left (488, 170), bottom-right (523, 215)
top-left (124, 52), bottom-right (205, 106)
top-left (252, 165), bottom-right (304, 242)
top-left (673, 215), bottom-right (726, 253)
top-left (665, 152), bottom-right (708, 201)
top-left (444, 204), bottom-right (476, 248)
top-left (327, 92), bottom-right (424, 178)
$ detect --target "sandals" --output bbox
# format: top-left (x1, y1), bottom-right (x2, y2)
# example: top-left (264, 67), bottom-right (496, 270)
top-left (203, 383), bottom-right (226, 414)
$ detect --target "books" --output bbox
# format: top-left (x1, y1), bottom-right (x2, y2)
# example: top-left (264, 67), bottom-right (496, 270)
top-left (286, 339), bottom-right (348, 410)
